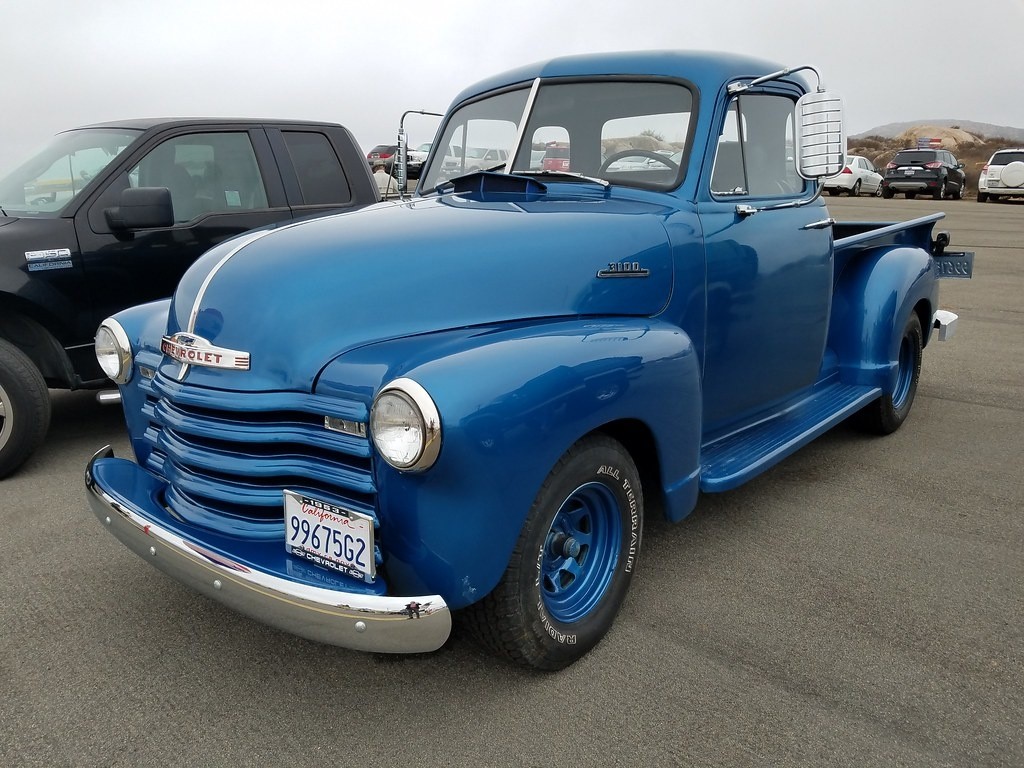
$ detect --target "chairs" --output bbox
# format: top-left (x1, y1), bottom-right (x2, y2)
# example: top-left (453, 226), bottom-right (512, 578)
top-left (711, 140), bottom-right (770, 195)
top-left (144, 162), bottom-right (196, 220)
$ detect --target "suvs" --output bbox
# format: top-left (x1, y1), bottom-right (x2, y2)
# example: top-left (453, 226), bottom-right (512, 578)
top-left (881, 147), bottom-right (966, 200)
top-left (976, 147), bottom-right (1024, 202)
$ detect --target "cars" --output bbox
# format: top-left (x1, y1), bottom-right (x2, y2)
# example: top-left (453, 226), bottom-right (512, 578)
top-left (824, 154), bottom-right (884, 197)
top-left (784, 146), bottom-right (798, 191)
top-left (365, 141), bottom-right (684, 182)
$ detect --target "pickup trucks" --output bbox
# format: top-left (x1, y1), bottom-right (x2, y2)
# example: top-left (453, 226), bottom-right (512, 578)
top-left (75, 44), bottom-right (978, 674)
top-left (0, 116), bottom-right (415, 481)
